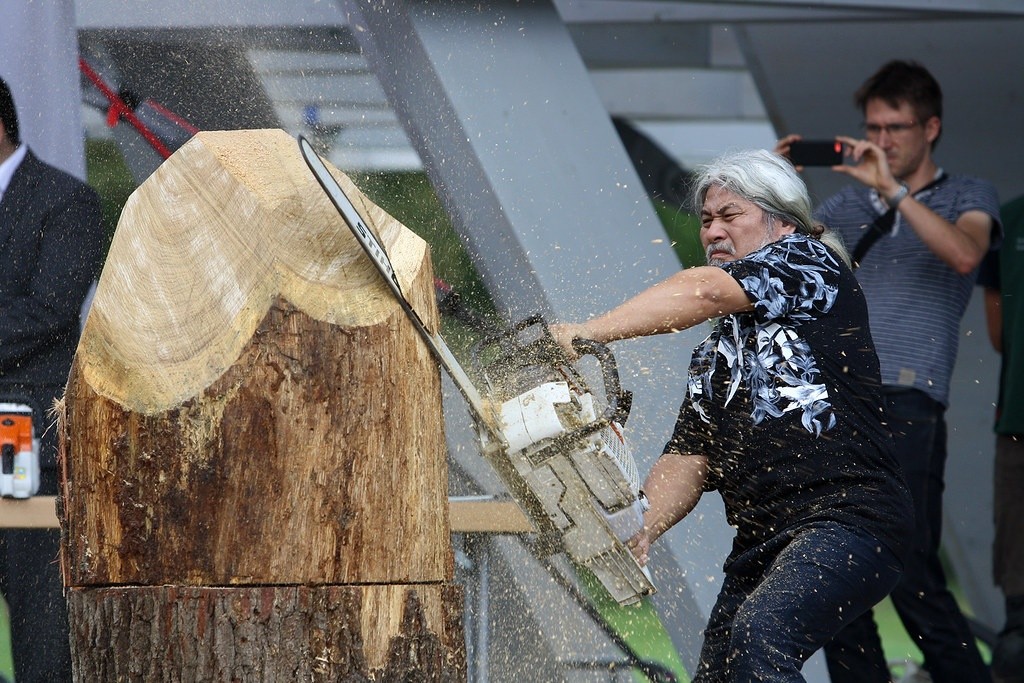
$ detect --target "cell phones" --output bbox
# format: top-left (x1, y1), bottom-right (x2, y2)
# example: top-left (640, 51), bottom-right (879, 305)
top-left (788, 139), bottom-right (844, 168)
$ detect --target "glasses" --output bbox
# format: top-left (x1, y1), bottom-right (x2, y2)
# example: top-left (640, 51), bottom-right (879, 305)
top-left (866, 120), bottom-right (926, 135)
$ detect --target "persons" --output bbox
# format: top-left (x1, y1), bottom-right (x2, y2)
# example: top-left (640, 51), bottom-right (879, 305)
top-left (977, 192), bottom-right (1024, 683)
top-left (545, 150), bottom-right (917, 683)
top-left (775, 59), bottom-right (1005, 683)
top-left (0, 79), bottom-right (103, 683)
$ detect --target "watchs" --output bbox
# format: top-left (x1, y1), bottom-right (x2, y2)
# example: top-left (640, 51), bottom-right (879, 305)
top-left (888, 180), bottom-right (910, 207)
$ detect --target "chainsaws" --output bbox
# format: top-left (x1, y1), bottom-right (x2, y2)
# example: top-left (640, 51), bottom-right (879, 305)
top-left (296, 134), bottom-right (658, 609)
